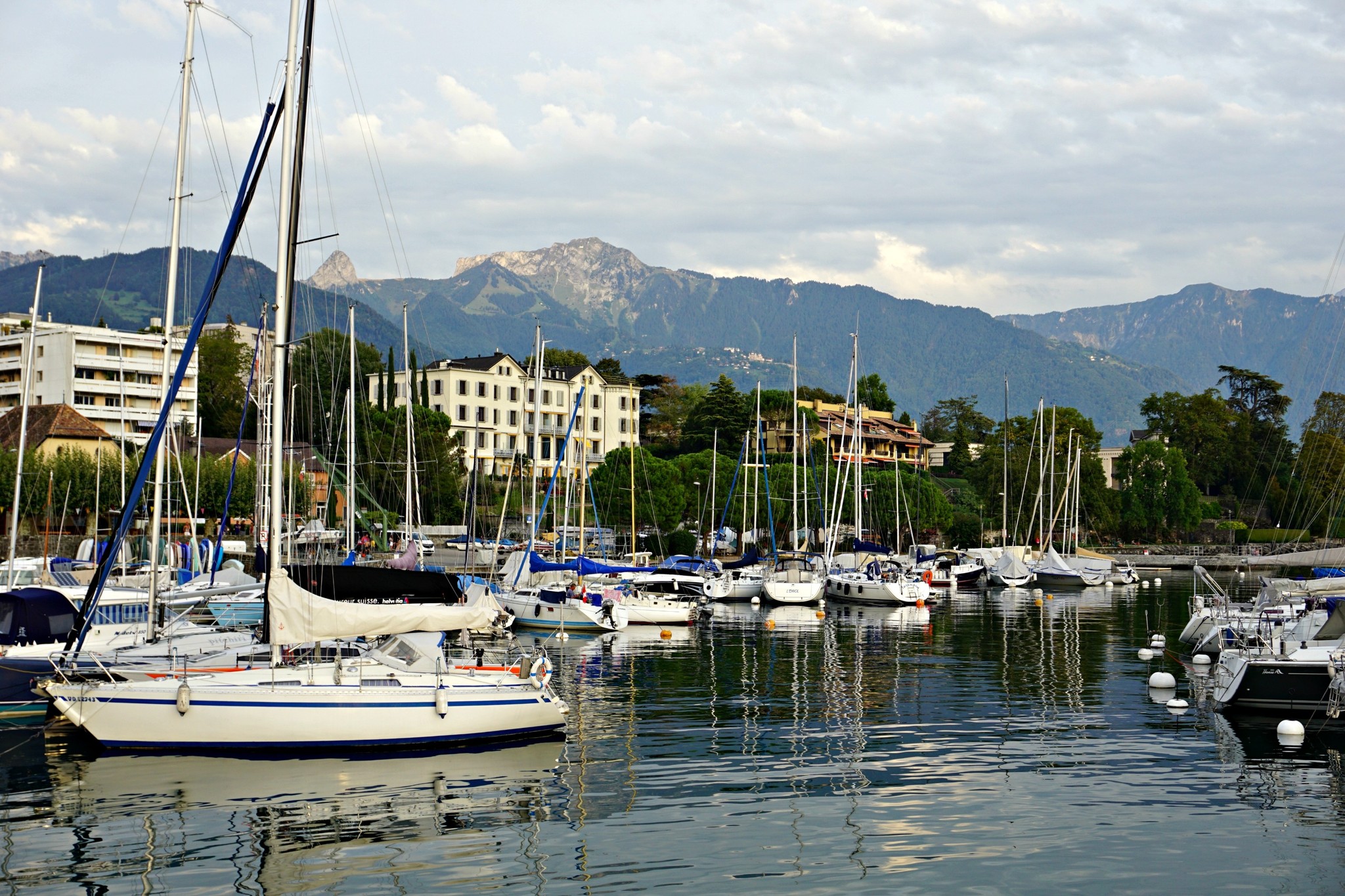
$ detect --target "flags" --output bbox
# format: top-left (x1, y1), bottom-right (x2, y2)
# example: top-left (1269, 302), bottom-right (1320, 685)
top-left (864, 489), bottom-right (868, 502)
top-left (582, 585), bottom-right (587, 603)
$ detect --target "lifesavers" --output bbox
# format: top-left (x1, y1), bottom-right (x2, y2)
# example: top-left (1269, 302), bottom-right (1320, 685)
top-left (1327, 651), bottom-right (1345, 680)
top-left (529, 657), bottom-right (553, 689)
top-left (923, 570), bottom-right (932, 583)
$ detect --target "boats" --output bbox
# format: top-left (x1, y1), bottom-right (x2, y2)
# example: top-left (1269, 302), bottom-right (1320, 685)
top-left (0, 0), bottom-right (1343, 772)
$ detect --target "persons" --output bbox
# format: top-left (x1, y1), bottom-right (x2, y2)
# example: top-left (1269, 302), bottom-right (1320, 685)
top-left (1036, 531), bottom-right (1142, 548)
top-left (1255, 548), bottom-right (1259, 555)
top-left (1251, 547), bottom-right (1255, 555)
top-left (354, 532), bottom-right (402, 555)
top-left (181, 514), bottom-right (256, 539)
top-left (1232, 546), bottom-right (1235, 553)
top-left (567, 583), bottom-right (579, 599)
